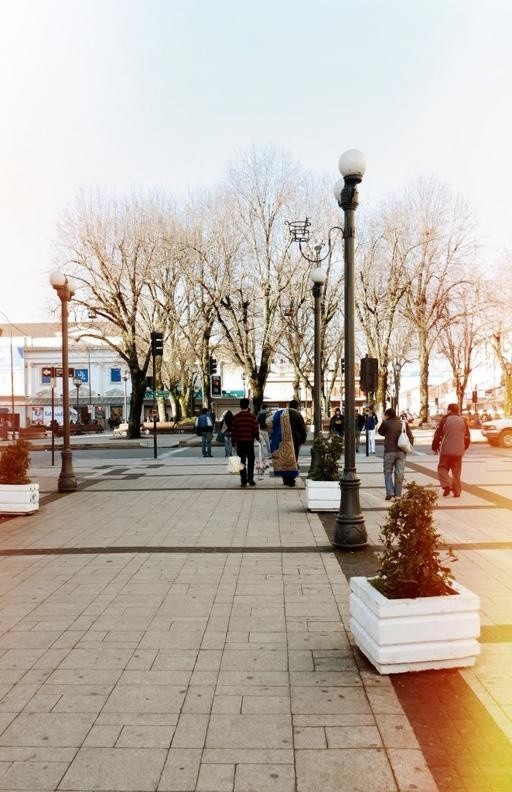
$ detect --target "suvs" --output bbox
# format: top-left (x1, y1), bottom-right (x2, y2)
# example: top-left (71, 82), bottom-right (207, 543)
top-left (480, 416), bottom-right (512, 449)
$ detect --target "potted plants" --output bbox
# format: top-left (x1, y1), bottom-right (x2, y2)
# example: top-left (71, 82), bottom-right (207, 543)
top-left (305, 430), bottom-right (347, 512)
top-left (347, 479), bottom-right (480, 677)
top-left (0, 438), bottom-right (39, 517)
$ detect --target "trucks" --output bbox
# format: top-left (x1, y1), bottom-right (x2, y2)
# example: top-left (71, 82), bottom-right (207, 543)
top-left (31, 405), bottom-right (79, 425)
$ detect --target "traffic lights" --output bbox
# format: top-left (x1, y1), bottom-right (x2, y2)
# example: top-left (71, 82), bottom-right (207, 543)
top-left (150, 330), bottom-right (164, 356)
top-left (341, 357), bottom-right (344, 374)
top-left (209, 355), bottom-right (217, 374)
top-left (210, 375), bottom-right (222, 399)
top-left (472, 391), bottom-right (477, 403)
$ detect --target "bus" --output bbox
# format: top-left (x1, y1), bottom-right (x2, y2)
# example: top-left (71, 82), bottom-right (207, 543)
top-left (310, 396), bottom-right (390, 432)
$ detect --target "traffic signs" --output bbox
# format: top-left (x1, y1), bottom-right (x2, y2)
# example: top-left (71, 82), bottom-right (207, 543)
top-left (154, 390), bottom-right (170, 398)
top-left (55, 367), bottom-right (75, 377)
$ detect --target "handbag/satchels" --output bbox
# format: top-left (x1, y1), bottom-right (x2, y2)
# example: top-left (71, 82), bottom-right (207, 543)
top-left (221, 423), bottom-right (227, 433)
top-left (398, 433), bottom-right (412, 453)
top-left (216, 433), bottom-right (224, 442)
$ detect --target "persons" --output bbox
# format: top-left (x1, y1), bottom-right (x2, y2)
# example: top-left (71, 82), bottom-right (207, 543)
top-left (230, 398), bottom-right (261, 487)
top-left (365, 409), bottom-right (378, 454)
top-left (257, 405), bottom-right (273, 455)
top-left (354, 409), bottom-right (365, 453)
top-left (195, 408), bottom-right (214, 456)
top-left (405, 409), bottom-right (414, 425)
top-left (431, 404), bottom-right (470, 497)
top-left (219, 410), bottom-right (235, 456)
top-left (378, 407), bottom-right (414, 499)
top-left (281, 399), bottom-right (307, 487)
top-left (329, 408), bottom-right (345, 438)
top-left (400, 410), bottom-right (407, 421)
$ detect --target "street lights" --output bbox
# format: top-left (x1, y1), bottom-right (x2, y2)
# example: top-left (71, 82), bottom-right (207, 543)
top-left (73, 378), bottom-right (82, 434)
top-left (50, 271), bottom-right (77, 492)
top-left (123, 375), bottom-right (129, 424)
top-left (309, 268), bottom-right (326, 481)
top-left (330, 148), bottom-right (371, 550)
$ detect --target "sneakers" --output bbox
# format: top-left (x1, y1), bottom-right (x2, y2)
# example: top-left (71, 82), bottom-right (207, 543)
top-left (443, 487), bottom-right (451, 496)
top-left (385, 495), bottom-right (394, 500)
top-left (240, 479), bottom-right (255, 487)
